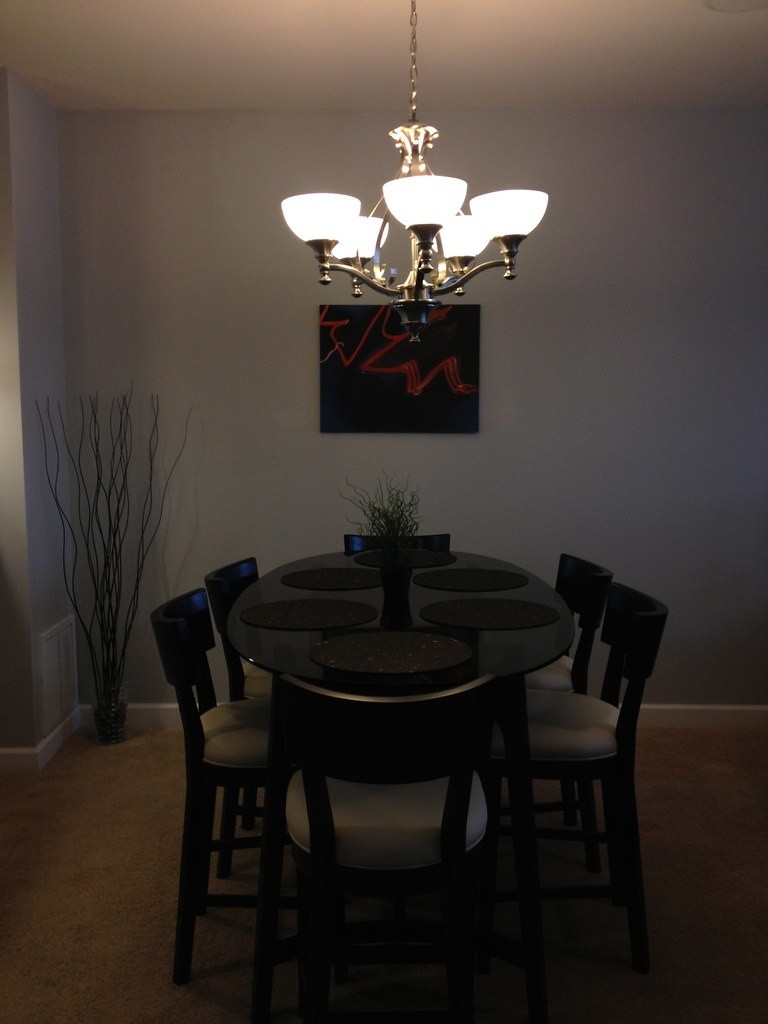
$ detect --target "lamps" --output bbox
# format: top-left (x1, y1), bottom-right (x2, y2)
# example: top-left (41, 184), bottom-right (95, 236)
top-left (281, 0), bottom-right (549, 344)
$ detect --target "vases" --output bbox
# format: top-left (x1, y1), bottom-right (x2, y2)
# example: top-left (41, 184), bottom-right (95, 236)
top-left (88, 681), bottom-right (129, 744)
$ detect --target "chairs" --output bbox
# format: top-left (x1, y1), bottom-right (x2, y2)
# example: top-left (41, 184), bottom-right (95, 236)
top-left (149, 588), bottom-right (271, 986)
top-left (344, 534), bottom-right (450, 550)
top-left (474, 581), bottom-right (669, 971)
top-left (205, 556), bottom-right (273, 879)
top-left (277, 673), bottom-right (494, 1024)
top-left (497, 553), bottom-right (613, 872)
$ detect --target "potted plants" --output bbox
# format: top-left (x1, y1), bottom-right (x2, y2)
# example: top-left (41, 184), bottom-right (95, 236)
top-left (337, 472), bottom-right (425, 628)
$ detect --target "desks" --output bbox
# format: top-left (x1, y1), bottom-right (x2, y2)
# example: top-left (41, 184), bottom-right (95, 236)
top-left (226, 549), bottom-right (576, 1024)
